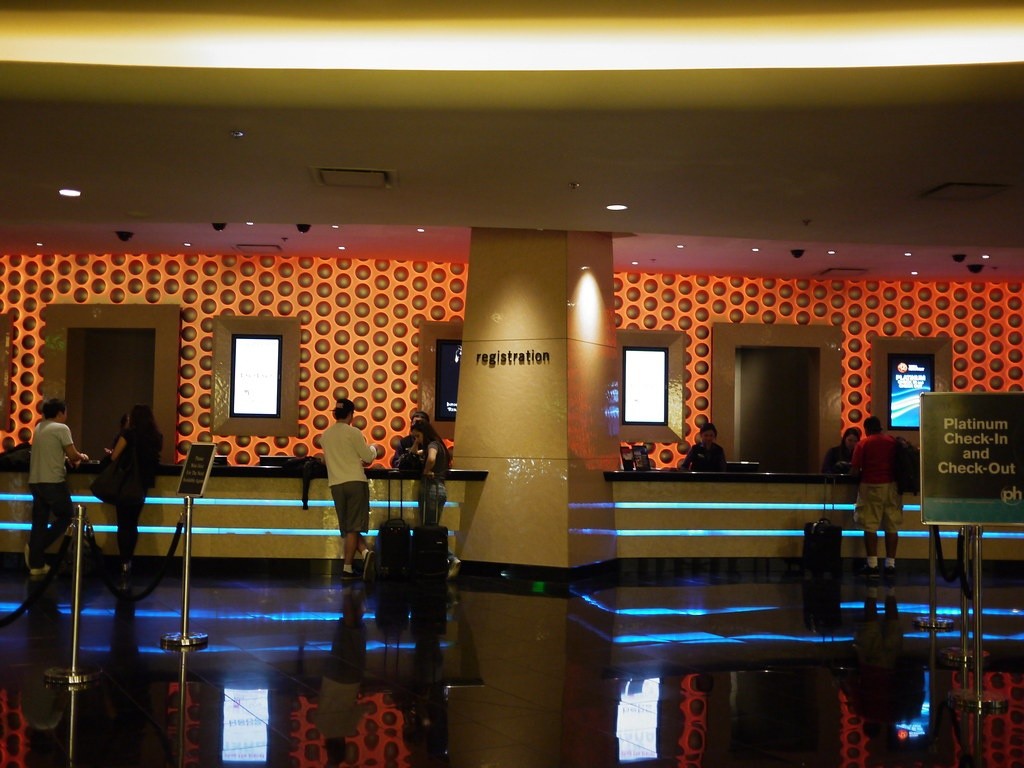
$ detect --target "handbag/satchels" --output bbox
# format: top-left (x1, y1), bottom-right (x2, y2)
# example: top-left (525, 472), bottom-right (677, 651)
top-left (398, 453), bottom-right (423, 473)
top-left (0, 444), bottom-right (30, 472)
top-left (91, 461), bottom-right (132, 506)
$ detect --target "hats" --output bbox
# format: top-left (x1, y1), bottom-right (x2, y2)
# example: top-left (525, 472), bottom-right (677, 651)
top-left (337, 398), bottom-right (355, 409)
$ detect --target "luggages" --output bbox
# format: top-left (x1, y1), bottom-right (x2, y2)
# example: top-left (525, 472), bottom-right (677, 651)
top-left (375, 586), bottom-right (410, 682)
top-left (410, 576), bottom-right (448, 636)
top-left (378, 471), bottom-right (412, 584)
top-left (803, 475), bottom-right (843, 573)
top-left (414, 475), bottom-right (448, 579)
top-left (803, 570), bottom-right (841, 634)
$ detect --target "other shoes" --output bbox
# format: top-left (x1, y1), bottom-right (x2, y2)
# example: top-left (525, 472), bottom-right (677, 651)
top-left (339, 571), bottom-right (363, 580)
top-left (446, 560), bottom-right (461, 582)
top-left (886, 567), bottom-right (905, 577)
top-left (24, 543), bottom-right (50, 575)
top-left (363, 551), bottom-right (376, 583)
top-left (119, 560), bottom-right (133, 577)
top-left (854, 562), bottom-right (882, 577)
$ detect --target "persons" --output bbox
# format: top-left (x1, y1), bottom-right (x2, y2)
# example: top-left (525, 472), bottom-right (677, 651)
top-left (320, 401), bottom-right (377, 584)
top-left (844, 418), bottom-right (906, 577)
top-left (822, 430), bottom-right (857, 475)
top-left (392, 411), bottom-right (462, 581)
top-left (22, 399), bottom-right (89, 577)
top-left (108, 404), bottom-right (157, 576)
top-left (681, 424), bottom-right (727, 472)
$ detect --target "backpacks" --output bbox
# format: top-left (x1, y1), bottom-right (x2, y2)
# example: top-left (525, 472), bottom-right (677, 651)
top-left (894, 437), bottom-right (921, 496)
